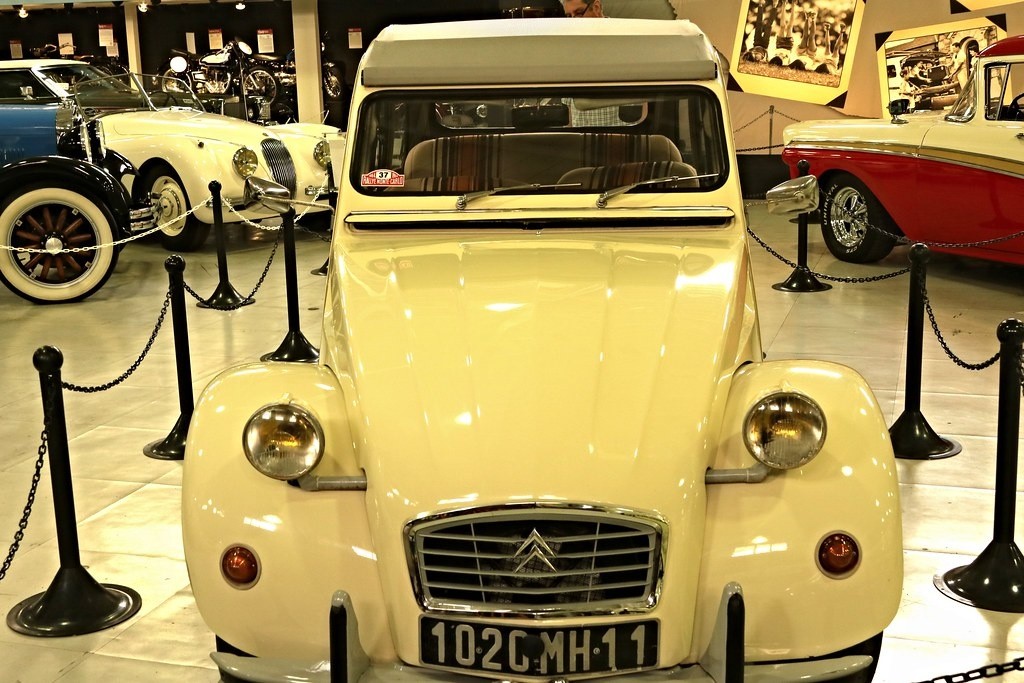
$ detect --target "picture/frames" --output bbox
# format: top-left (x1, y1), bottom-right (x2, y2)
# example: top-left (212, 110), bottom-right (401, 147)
top-left (875, 13), bottom-right (1011, 120)
top-left (725, 0), bottom-right (865, 108)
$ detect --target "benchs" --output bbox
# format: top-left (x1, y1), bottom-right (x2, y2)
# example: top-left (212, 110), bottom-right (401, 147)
top-left (384, 132), bottom-right (700, 193)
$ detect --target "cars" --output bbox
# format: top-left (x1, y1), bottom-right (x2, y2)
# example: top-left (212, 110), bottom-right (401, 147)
top-left (1, 41), bottom-right (349, 306)
top-left (178, 14), bottom-right (910, 683)
top-left (777, 32), bottom-right (1023, 270)
